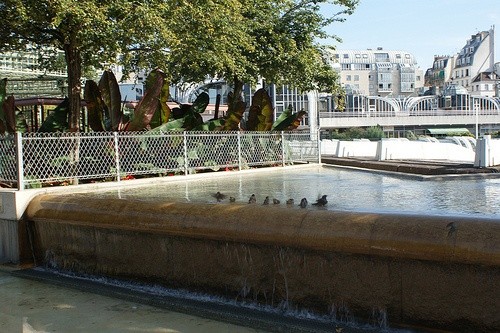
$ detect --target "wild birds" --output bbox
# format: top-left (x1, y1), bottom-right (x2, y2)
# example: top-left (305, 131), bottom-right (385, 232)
top-left (229, 196), bottom-right (235, 202)
top-left (286, 198), bottom-right (295, 205)
top-left (311, 195), bottom-right (328, 208)
top-left (249, 194), bottom-right (256, 204)
top-left (273, 198), bottom-right (280, 204)
top-left (262, 196), bottom-right (269, 206)
top-left (298, 198), bottom-right (307, 208)
top-left (211, 191), bottom-right (227, 201)
